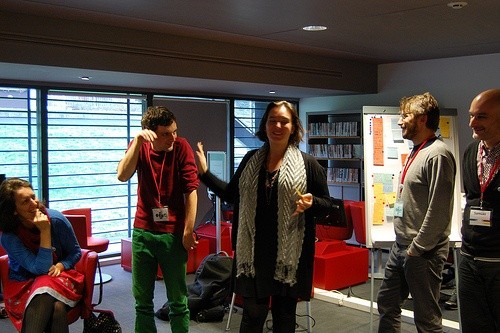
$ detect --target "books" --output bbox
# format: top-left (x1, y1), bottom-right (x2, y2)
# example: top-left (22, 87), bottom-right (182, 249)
top-left (308, 144), bottom-right (317, 157)
top-left (337, 121), bottom-right (360, 136)
top-left (309, 123), bottom-right (336, 136)
top-left (317, 144), bottom-right (337, 158)
top-left (327, 168), bottom-right (344, 183)
top-left (344, 169), bottom-right (360, 183)
top-left (337, 144), bottom-right (361, 158)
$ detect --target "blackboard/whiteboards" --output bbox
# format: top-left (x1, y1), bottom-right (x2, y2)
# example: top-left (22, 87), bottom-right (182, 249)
top-left (362, 105), bottom-right (463, 247)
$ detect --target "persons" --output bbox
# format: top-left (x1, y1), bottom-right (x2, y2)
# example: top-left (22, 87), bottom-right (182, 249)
top-left (377, 92), bottom-right (457, 333)
top-left (117, 105), bottom-right (200, 333)
top-left (0, 178), bottom-right (85, 333)
top-left (458, 89), bottom-right (500, 333)
top-left (195, 99), bottom-right (333, 333)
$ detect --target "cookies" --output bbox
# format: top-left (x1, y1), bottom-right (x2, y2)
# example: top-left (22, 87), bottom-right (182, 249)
top-left (295, 186), bottom-right (304, 198)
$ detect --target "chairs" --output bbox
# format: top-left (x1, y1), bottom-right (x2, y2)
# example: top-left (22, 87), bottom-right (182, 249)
top-left (121, 198), bottom-right (364, 332)
top-left (0, 209), bottom-right (109, 333)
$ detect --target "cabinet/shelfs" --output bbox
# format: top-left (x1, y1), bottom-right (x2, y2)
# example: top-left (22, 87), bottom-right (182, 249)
top-left (305, 109), bottom-right (363, 202)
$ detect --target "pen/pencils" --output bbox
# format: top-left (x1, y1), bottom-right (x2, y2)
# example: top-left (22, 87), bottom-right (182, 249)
top-left (294, 188), bottom-right (302, 197)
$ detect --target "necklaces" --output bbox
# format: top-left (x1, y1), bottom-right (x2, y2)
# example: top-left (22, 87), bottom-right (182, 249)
top-left (264, 157), bottom-right (281, 205)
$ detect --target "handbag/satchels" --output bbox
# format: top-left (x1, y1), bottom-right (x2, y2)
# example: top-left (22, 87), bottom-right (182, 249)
top-left (159, 249), bottom-right (236, 321)
top-left (82, 311), bottom-right (122, 333)
